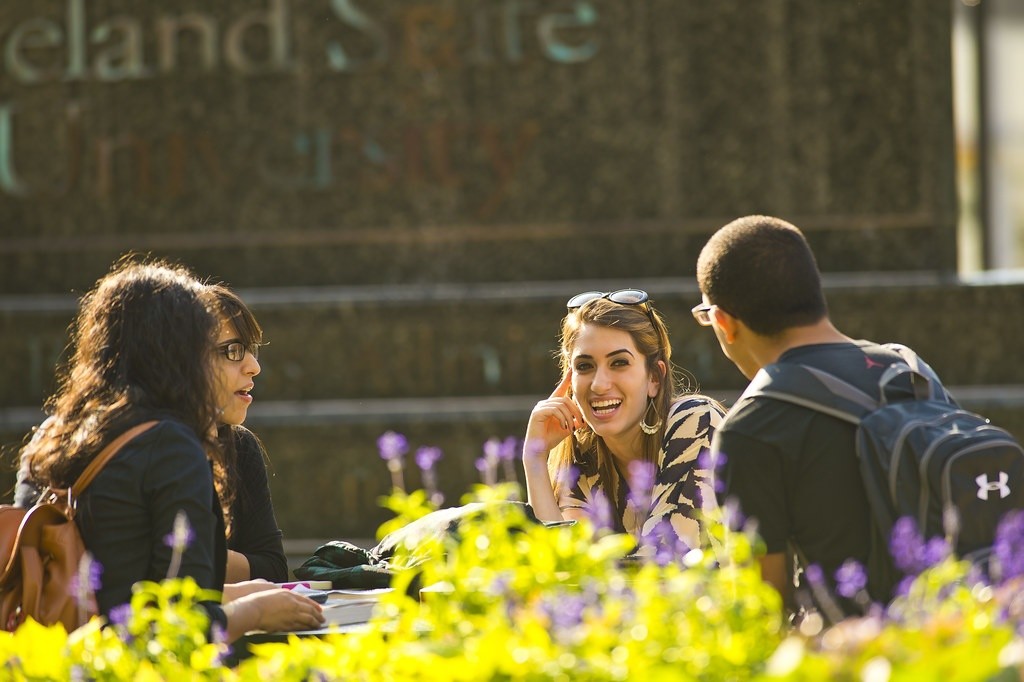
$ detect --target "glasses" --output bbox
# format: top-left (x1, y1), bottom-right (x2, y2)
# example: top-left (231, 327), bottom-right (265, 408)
top-left (692, 302), bottom-right (729, 326)
top-left (566, 288), bottom-right (667, 363)
top-left (211, 339), bottom-right (260, 362)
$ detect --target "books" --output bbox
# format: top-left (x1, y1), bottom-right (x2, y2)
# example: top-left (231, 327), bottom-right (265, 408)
top-left (240, 584), bottom-right (394, 633)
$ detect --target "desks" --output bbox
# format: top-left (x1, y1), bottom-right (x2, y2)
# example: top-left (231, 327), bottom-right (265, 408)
top-left (241, 621), bottom-right (367, 643)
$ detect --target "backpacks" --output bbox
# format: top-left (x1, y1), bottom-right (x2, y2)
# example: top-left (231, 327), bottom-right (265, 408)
top-left (749, 339), bottom-right (1024, 608)
top-left (0, 419), bottom-right (162, 636)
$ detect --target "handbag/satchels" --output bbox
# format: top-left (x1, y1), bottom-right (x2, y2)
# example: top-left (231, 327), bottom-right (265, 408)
top-left (294, 500), bottom-right (574, 600)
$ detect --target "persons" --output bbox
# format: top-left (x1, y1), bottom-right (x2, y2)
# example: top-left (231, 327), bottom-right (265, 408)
top-left (10, 251), bottom-right (326, 657)
top-left (689, 211), bottom-right (987, 652)
top-left (198, 284), bottom-right (293, 585)
top-left (521, 288), bottom-right (730, 575)
